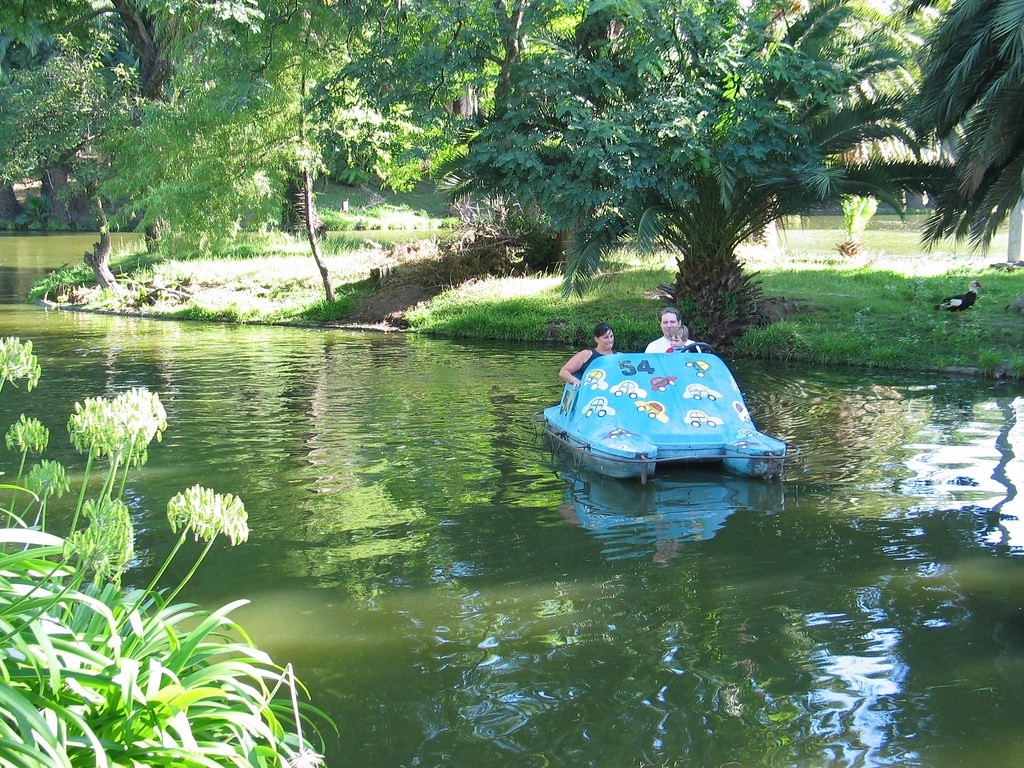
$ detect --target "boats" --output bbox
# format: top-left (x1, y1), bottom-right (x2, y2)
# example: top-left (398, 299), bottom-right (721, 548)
top-left (529, 342), bottom-right (803, 484)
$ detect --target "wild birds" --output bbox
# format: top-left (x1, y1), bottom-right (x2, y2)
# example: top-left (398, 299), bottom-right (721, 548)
top-left (933, 280), bottom-right (982, 318)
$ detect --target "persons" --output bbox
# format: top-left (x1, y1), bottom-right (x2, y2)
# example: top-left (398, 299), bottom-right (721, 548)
top-left (665, 325), bottom-right (689, 354)
top-left (645, 307), bottom-right (702, 353)
top-left (559, 323), bottom-right (617, 390)
top-left (934, 280), bottom-right (981, 312)
